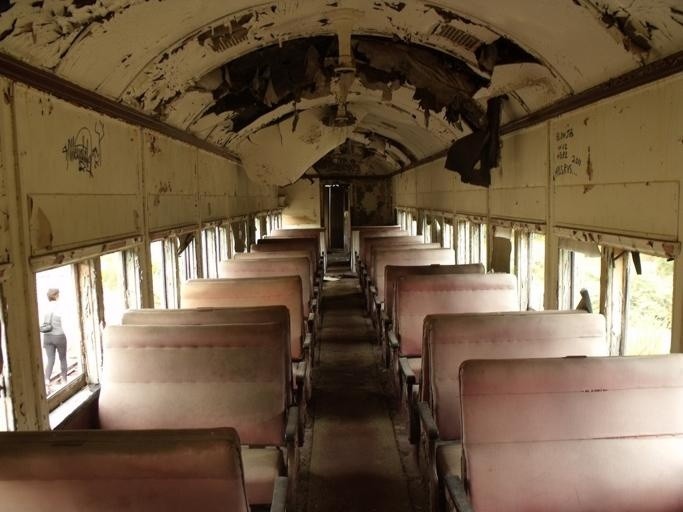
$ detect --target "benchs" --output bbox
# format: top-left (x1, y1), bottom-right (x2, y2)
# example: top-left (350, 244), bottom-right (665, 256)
top-left (1, 227), bottom-right (326, 511)
top-left (350, 224), bottom-right (683, 511)
